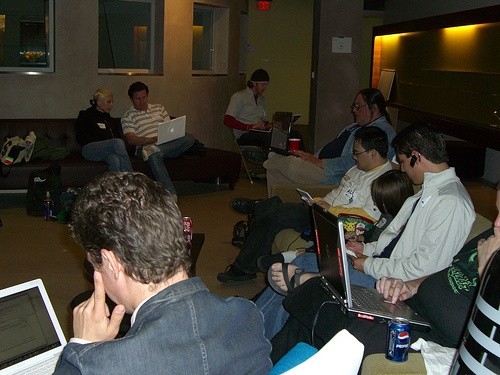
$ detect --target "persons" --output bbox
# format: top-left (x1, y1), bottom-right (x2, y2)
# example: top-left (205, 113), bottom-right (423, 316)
top-left (257, 123), bottom-right (478, 344)
top-left (269, 182), bottom-right (500, 368)
top-left (76, 88), bottom-right (136, 171)
top-left (224, 68), bottom-right (308, 159)
top-left (122, 81), bottom-right (197, 202)
top-left (244, 86), bottom-right (397, 203)
top-left (226, 171), bottom-right (415, 304)
top-left (216, 125), bottom-right (394, 282)
top-left (53, 169), bottom-right (275, 374)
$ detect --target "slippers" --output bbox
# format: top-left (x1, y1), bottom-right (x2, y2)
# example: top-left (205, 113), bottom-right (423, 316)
top-left (267, 263), bottom-right (322, 295)
top-left (257, 250), bottom-right (294, 272)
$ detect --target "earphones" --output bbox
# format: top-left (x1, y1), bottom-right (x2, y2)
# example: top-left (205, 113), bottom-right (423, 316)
top-left (410, 156), bottom-right (416, 168)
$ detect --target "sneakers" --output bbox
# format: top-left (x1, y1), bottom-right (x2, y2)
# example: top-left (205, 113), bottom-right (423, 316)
top-left (217, 270), bottom-right (257, 283)
top-left (231, 196), bottom-right (264, 212)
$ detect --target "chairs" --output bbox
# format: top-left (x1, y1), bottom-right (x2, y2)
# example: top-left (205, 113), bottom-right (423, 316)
top-left (232, 130), bottom-right (269, 183)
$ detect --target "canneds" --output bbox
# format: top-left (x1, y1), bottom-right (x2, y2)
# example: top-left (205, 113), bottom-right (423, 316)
top-left (183, 215), bottom-right (193, 245)
top-left (386, 317), bottom-right (412, 365)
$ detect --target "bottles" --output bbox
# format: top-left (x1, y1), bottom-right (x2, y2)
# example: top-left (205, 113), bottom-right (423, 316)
top-left (42, 192), bottom-right (54, 222)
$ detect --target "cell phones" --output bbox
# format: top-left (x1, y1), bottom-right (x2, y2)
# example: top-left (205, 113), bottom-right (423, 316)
top-left (295, 188), bottom-right (313, 202)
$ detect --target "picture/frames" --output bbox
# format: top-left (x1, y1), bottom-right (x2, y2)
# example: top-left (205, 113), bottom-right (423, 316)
top-left (378, 70), bottom-right (394, 100)
top-left (386, 106), bottom-right (398, 130)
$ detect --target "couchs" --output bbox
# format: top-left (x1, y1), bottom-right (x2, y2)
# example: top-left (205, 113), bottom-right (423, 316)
top-left (268, 181), bottom-right (493, 375)
top-left (0, 117), bottom-right (220, 189)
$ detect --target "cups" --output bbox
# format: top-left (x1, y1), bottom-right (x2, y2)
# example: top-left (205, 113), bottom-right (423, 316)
top-left (289, 139), bottom-right (301, 151)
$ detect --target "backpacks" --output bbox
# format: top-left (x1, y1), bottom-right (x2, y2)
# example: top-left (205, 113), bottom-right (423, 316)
top-left (27, 166), bottom-right (63, 218)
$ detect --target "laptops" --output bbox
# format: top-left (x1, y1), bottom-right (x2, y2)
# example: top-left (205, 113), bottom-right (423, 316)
top-left (310, 203), bottom-right (432, 333)
top-left (0, 279), bottom-right (67, 375)
top-left (156, 115), bottom-right (187, 145)
top-left (250, 111), bottom-right (307, 160)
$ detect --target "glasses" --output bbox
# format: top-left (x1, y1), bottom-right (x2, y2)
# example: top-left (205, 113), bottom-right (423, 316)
top-left (351, 104), bottom-right (369, 110)
top-left (352, 148), bottom-right (372, 157)
